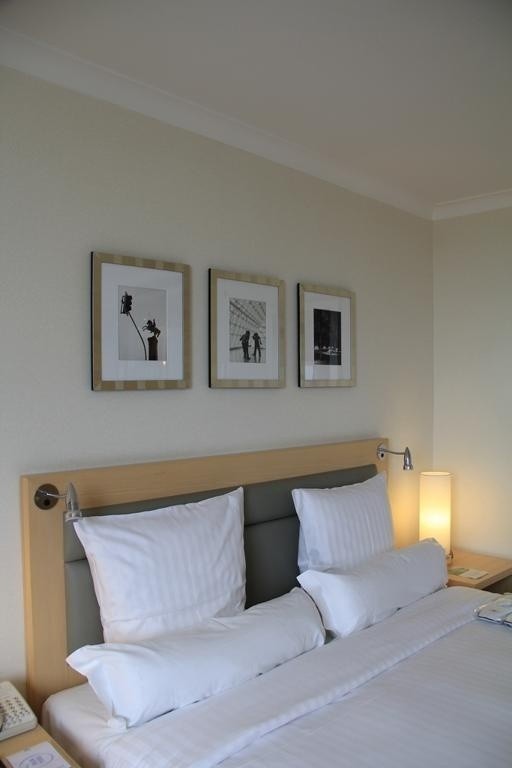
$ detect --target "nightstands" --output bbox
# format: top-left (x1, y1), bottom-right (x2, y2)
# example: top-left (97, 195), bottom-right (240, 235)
top-left (446, 550), bottom-right (512, 595)
top-left (0, 725), bottom-right (83, 768)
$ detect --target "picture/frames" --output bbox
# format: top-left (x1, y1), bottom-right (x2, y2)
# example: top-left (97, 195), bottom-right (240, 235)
top-left (297, 281), bottom-right (357, 388)
top-left (92, 250), bottom-right (192, 391)
top-left (208, 267), bottom-right (287, 390)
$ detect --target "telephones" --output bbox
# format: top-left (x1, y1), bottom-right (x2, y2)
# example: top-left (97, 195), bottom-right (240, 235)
top-left (0, 678), bottom-right (39, 741)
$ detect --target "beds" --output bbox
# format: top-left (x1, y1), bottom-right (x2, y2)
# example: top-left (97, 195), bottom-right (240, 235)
top-left (20, 438), bottom-right (512, 768)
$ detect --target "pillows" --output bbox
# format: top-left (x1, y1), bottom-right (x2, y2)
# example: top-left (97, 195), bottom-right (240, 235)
top-left (73, 487), bottom-right (247, 644)
top-left (296, 536), bottom-right (449, 639)
top-left (291, 470), bottom-right (396, 575)
top-left (65, 588), bottom-right (322, 731)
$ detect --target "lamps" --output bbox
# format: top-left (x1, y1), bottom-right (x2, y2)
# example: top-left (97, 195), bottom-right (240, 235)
top-left (377, 442), bottom-right (414, 471)
top-left (35, 482), bottom-right (81, 523)
top-left (419, 470), bottom-right (452, 556)
